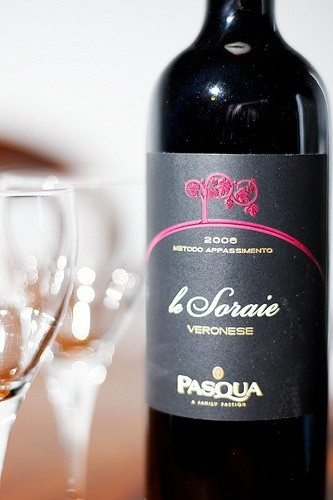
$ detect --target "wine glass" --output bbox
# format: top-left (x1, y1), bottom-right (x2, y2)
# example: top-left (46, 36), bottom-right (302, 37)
top-left (1, 174), bottom-right (80, 477)
top-left (42, 178), bottom-right (147, 500)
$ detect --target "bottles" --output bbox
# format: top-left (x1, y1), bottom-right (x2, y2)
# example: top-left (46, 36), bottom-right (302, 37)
top-left (145, 0), bottom-right (330, 500)
